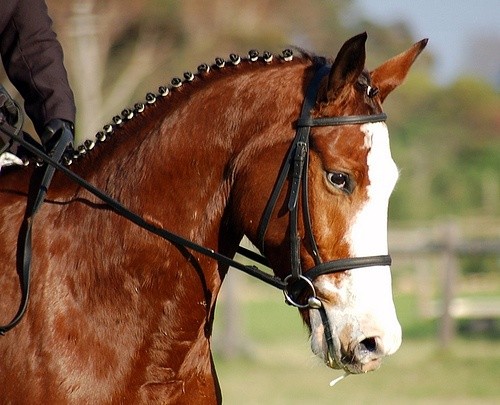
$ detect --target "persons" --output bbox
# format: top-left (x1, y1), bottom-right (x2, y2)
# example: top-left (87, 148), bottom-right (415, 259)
top-left (0, 0), bottom-right (79, 169)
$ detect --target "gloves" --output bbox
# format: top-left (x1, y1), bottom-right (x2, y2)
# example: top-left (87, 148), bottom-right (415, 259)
top-left (0, 86), bottom-right (18, 127)
top-left (44, 120), bottom-right (73, 156)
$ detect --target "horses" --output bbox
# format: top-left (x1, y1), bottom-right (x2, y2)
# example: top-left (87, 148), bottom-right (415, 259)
top-left (0, 31), bottom-right (429, 405)
